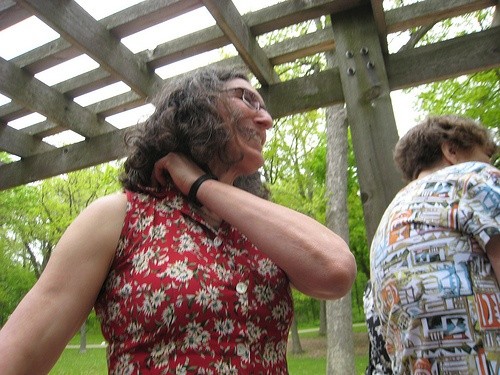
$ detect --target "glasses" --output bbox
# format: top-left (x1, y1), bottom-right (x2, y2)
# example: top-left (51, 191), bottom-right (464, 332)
top-left (224, 88), bottom-right (266, 111)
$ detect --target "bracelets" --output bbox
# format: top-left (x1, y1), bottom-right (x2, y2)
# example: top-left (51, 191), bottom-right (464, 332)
top-left (186, 174), bottom-right (221, 208)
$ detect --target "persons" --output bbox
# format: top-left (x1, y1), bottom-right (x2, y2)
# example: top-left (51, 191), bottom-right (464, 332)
top-left (0, 65), bottom-right (357, 375)
top-left (363, 280), bottom-right (393, 375)
top-left (370, 116), bottom-right (500, 375)
